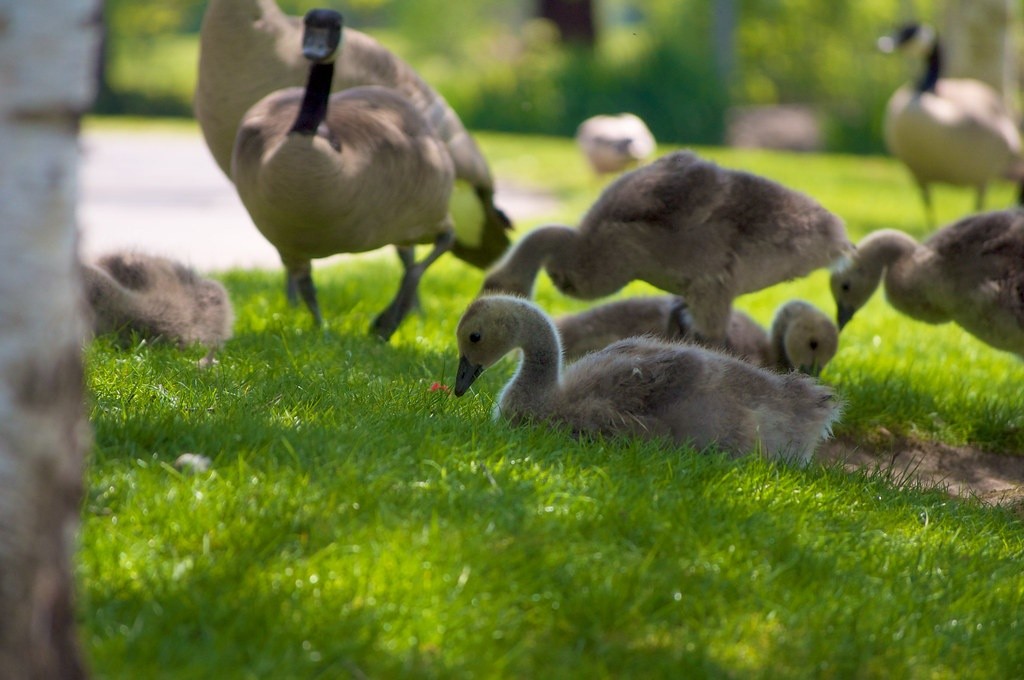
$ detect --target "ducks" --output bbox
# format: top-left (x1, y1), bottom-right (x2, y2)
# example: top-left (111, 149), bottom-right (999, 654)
top-left (78, 1), bottom-right (1024, 475)
